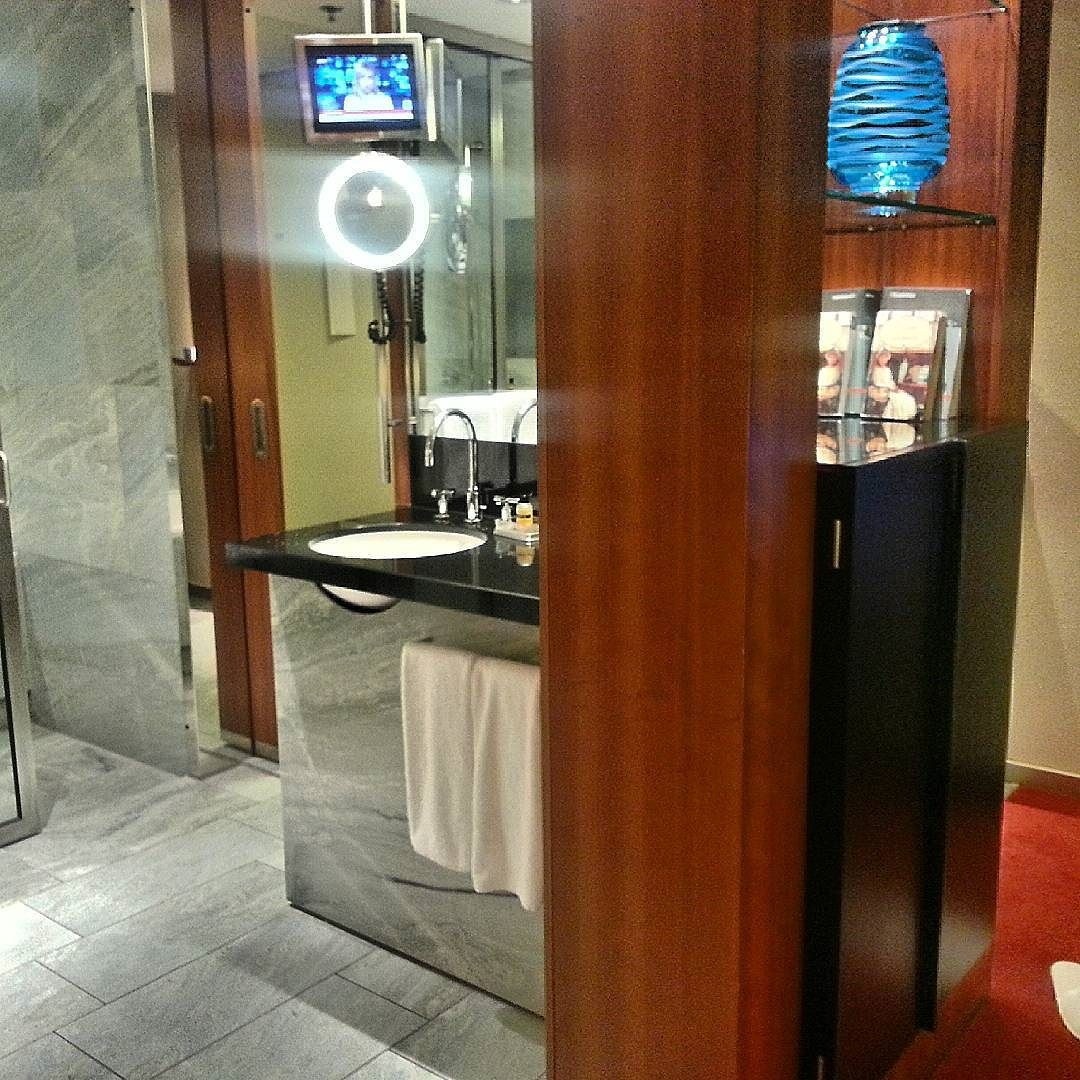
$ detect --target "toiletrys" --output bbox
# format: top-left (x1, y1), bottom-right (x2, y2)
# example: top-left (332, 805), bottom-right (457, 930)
top-left (517, 493), bottom-right (535, 528)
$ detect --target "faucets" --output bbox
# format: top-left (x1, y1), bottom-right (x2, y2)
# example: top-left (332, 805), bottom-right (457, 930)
top-left (422, 407), bottom-right (488, 526)
top-left (508, 399), bottom-right (538, 491)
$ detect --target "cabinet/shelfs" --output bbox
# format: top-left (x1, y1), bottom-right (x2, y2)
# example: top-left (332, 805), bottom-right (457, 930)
top-left (798, 442), bottom-right (920, 1080)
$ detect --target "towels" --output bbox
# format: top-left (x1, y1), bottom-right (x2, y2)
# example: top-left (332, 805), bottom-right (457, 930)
top-left (398, 640), bottom-right (547, 913)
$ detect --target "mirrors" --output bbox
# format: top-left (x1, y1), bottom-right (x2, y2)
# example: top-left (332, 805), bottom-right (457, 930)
top-left (399, 0), bottom-right (537, 397)
top-left (815, 228), bottom-right (896, 420)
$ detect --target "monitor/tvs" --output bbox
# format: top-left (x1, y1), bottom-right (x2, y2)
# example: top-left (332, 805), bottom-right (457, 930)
top-left (293, 32), bottom-right (438, 143)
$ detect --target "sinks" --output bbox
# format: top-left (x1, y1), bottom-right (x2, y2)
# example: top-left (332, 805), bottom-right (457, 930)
top-left (306, 523), bottom-right (491, 562)
